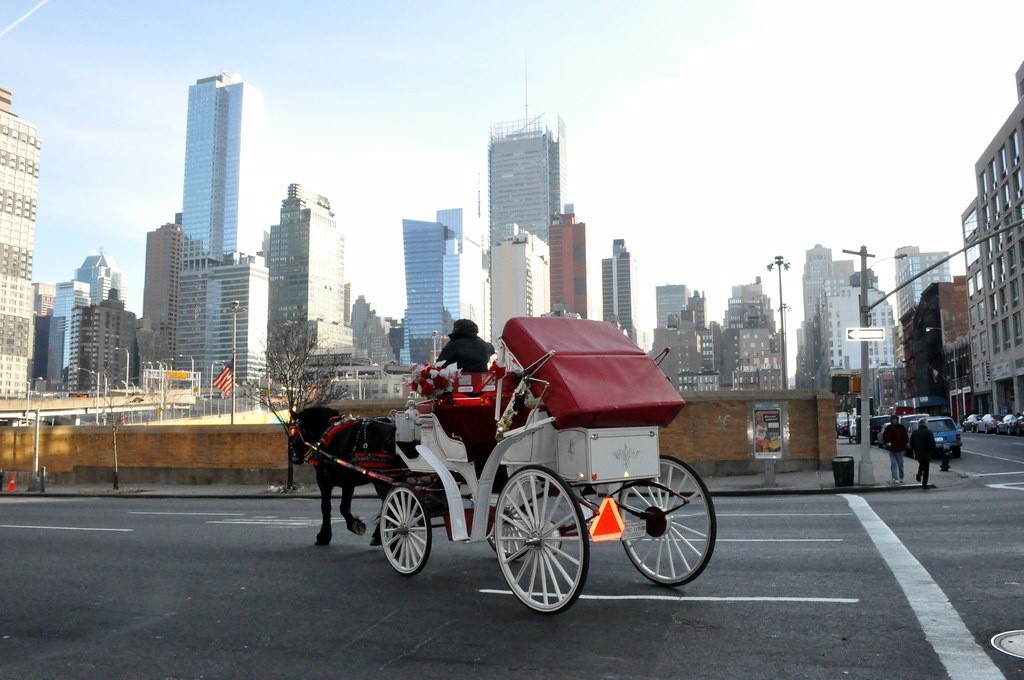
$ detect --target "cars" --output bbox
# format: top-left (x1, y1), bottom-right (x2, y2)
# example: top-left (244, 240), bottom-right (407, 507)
top-left (961, 414), bottom-right (984, 433)
top-left (836, 411), bottom-right (962, 460)
top-left (996, 413), bottom-right (1024, 436)
top-left (975, 414), bottom-right (1007, 434)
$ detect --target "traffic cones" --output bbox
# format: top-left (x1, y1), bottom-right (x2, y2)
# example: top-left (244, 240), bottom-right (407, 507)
top-left (3, 472), bottom-right (20, 492)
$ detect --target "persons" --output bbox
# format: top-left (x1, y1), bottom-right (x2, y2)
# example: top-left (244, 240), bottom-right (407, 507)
top-left (909, 419), bottom-right (936, 489)
top-left (437, 319), bottom-right (495, 397)
top-left (882, 415), bottom-right (909, 484)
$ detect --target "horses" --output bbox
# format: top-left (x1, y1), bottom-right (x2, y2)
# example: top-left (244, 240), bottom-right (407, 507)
top-left (290, 406), bottom-right (424, 559)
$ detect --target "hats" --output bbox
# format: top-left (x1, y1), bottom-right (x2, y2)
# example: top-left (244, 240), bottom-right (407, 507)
top-left (453, 319), bottom-right (478, 332)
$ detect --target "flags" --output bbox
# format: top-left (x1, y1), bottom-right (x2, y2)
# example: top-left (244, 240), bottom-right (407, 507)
top-left (213, 358), bottom-right (234, 397)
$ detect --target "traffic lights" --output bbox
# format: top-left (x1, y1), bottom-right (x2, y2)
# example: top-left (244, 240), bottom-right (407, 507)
top-left (831, 375), bottom-right (850, 394)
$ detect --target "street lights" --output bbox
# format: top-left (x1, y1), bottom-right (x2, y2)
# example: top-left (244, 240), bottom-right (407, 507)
top-left (77, 366), bottom-right (100, 426)
top-left (115, 347), bottom-right (130, 397)
top-left (179, 354), bottom-right (194, 397)
top-left (767, 254), bottom-right (790, 390)
top-left (925, 327), bottom-right (960, 424)
top-left (230, 300), bottom-right (239, 424)
top-left (26, 376), bottom-right (43, 426)
top-left (209, 361), bottom-right (218, 415)
top-left (142, 361), bottom-right (173, 391)
top-left (158, 357), bottom-right (174, 390)
top-left (92, 370), bottom-right (108, 425)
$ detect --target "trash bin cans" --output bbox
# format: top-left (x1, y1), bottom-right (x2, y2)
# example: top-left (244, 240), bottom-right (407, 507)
top-left (832, 456), bottom-right (854, 487)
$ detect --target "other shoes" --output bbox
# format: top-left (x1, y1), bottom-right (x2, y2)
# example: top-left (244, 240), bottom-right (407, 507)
top-left (901, 477), bottom-right (904, 483)
top-left (916, 474), bottom-right (921, 482)
top-left (888, 478), bottom-right (898, 484)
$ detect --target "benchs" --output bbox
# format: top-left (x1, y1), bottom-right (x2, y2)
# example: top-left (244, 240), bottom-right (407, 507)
top-left (431, 406), bottom-right (496, 462)
top-left (499, 410), bottom-right (559, 466)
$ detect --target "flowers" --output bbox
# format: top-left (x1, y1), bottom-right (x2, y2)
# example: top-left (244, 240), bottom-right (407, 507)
top-left (404, 359), bottom-right (463, 400)
top-left (495, 352), bottom-right (524, 376)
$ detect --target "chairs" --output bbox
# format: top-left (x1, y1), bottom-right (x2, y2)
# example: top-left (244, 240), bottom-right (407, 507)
top-left (414, 361), bottom-right (507, 415)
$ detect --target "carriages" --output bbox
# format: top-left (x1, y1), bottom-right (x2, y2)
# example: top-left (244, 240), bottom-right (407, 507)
top-left (282, 317), bottom-right (718, 615)
